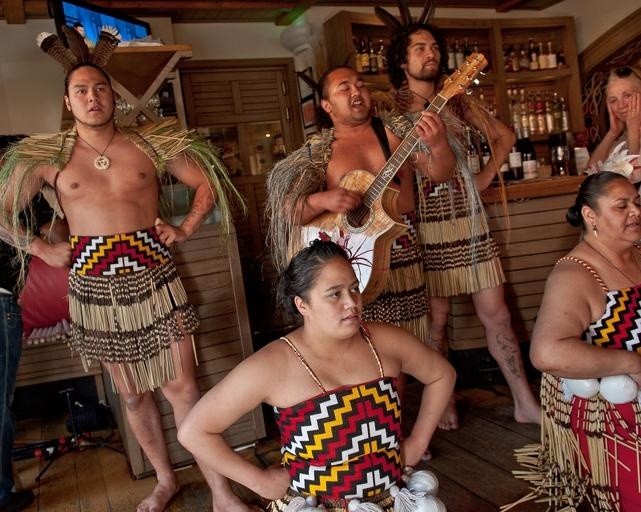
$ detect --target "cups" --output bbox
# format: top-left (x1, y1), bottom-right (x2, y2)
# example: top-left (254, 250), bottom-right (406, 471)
top-left (552, 146), bottom-right (570, 177)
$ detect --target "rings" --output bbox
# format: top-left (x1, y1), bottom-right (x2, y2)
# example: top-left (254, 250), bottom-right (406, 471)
top-left (632, 109), bottom-right (636, 112)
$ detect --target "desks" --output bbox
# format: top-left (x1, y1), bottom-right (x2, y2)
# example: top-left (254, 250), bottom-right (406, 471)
top-left (88, 44), bottom-right (193, 128)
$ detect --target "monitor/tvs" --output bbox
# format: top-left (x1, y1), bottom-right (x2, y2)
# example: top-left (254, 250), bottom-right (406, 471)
top-left (51, 1), bottom-right (151, 50)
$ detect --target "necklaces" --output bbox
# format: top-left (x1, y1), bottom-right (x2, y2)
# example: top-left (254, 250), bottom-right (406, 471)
top-left (582, 235), bottom-right (638, 289)
top-left (76, 130), bottom-right (116, 171)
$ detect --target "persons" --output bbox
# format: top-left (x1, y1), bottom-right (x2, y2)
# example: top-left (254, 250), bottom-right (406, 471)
top-left (385, 21), bottom-right (541, 435)
top-left (529, 171), bottom-right (641, 512)
top-left (177, 241), bottom-right (458, 512)
top-left (0, 134), bottom-right (56, 512)
top-left (586, 65), bottom-right (641, 170)
top-left (266, 67), bottom-right (458, 464)
top-left (0, 64), bottom-right (255, 512)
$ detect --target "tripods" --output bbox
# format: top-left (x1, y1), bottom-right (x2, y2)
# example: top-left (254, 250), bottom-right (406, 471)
top-left (35, 388), bottom-right (128, 482)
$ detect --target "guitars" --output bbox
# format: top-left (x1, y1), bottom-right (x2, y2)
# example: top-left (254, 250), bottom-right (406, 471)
top-left (285, 51), bottom-right (489, 307)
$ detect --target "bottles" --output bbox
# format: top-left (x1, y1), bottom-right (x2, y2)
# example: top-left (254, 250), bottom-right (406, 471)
top-left (352, 35), bottom-right (386, 73)
top-left (502, 37), bottom-right (566, 72)
top-left (467, 127), bottom-right (538, 184)
top-left (445, 37), bottom-right (480, 74)
top-left (507, 88), bottom-right (569, 135)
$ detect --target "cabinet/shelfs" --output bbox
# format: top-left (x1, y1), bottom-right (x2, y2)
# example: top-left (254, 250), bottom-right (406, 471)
top-left (101, 221), bottom-right (268, 481)
top-left (323, 10), bottom-right (591, 176)
